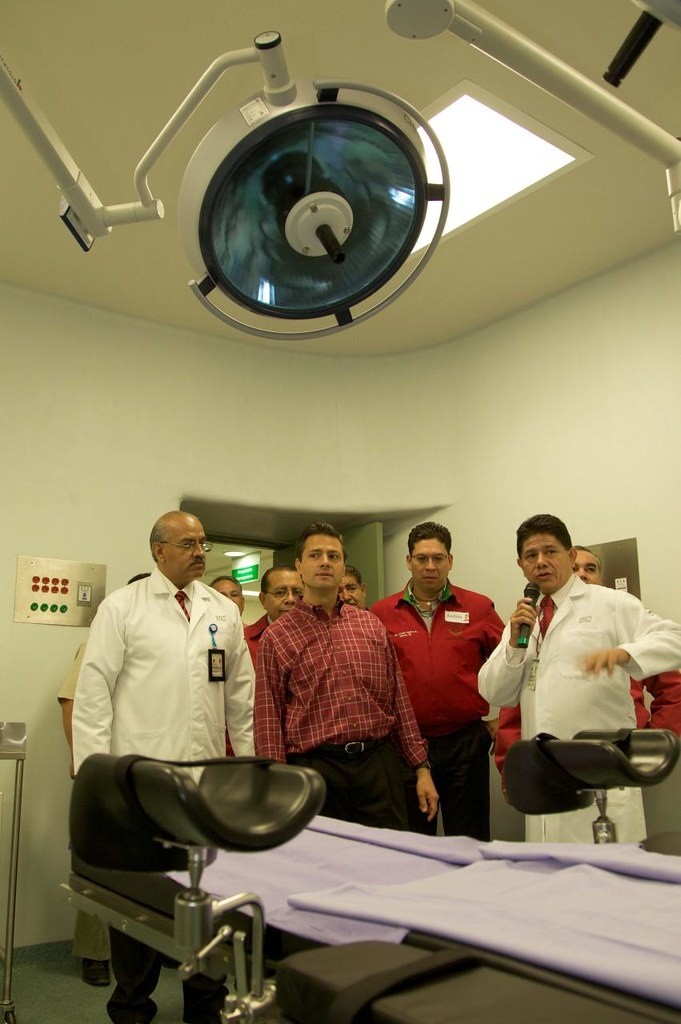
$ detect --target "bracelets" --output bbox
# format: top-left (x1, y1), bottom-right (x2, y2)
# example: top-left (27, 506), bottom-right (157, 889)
top-left (413, 760), bottom-right (431, 772)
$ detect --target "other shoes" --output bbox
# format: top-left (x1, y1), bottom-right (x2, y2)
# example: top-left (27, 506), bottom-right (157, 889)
top-left (82, 958), bottom-right (111, 986)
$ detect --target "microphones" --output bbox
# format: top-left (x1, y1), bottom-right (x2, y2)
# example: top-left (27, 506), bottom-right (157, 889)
top-left (517, 583), bottom-right (541, 649)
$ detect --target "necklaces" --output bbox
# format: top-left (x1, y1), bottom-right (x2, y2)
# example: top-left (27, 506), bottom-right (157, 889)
top-left (412, 592), bottom-right (440, 607)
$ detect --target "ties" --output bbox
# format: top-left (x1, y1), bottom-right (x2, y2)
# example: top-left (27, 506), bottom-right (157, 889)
top-left (540, 595), bottom-right (554, 639)
top-left (174, 591), bottom-right (190, 622)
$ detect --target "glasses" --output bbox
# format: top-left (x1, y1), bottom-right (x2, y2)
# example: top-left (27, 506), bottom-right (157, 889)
top-left (412, 554), bottom-right (449, 564)
top-left (160, 541), bottom-right (213, 552)
top-left (266, 589), bottom-right (304, 600)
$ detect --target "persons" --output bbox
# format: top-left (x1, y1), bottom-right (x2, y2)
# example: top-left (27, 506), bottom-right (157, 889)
top-left (338, 564), bottom-right (370, 613)
top-left (71, 510), bottom-right (257, 1024)
top-left (477, 514), bottom-right (681, 844)
top-left (211, 565), bottom-right (306, 757)
top-left (493, 546), bottom-right (681, 805)
top-left (252, 522), bottom-right (439, 832)
top-left (57, 574), bottom-right (155, 986)
top-left (369, 522), bottom-right (506, 842)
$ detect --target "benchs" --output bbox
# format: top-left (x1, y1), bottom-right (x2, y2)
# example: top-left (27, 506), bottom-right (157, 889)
top-left (59, 728), bottom-right (681, 1024)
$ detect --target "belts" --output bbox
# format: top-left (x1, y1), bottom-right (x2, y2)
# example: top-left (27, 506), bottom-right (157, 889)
top-left (305, 740), bottom-right (377, 761)
